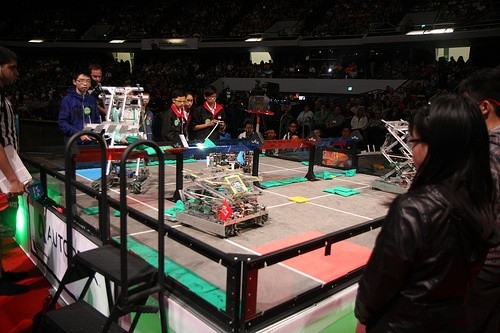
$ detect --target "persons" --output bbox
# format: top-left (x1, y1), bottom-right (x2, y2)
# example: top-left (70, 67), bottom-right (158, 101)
top-left (468, 91), bottom-right (500, 333)
top-left (88, 64), bottom-right (110, 147)
top-left (187, 84), bottom-right (226, 142)
top-left (353, 91), bottom-right (496, 333)
top-left (0, 0), bottom-right (500, 145)
top-left (0, 46), bottom-right (34, 296)
top-left (58, 69), bottom-right (102, 149)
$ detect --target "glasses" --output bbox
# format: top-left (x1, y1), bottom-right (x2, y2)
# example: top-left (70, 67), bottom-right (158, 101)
top-left (407, 136), bottom-right (424, 149)
top-left (75, 79), bottom-right (91, 84)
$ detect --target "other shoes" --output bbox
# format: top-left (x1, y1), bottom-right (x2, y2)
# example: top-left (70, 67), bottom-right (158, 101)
top-left (0, 282), bottom-right (30, 296)
top-left (2, 271), bottom-right (28, 282)
